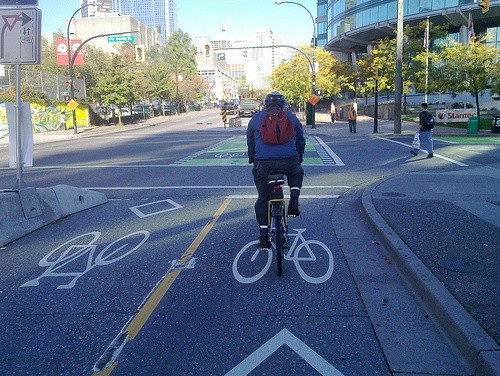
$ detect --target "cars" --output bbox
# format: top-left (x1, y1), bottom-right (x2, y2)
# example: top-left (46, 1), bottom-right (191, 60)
top-left (110, 109), bottom-right (137, 117)
top-left (238, 104), bottom-right (258, 118)
top-left (133, 105), bottom-right (148, 114)
top-left (220, 105), bottom-right (236, 115)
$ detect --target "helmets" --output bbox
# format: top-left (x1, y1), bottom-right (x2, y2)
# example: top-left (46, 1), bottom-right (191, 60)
top-left (265, 91), bottom-right (286, 107)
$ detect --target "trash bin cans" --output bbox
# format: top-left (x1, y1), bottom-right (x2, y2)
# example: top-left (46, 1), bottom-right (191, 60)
top-left (229, 117), bottom-right (241, 127)
top-left (189, 104), bottom-right (195, 111)
top-left (466, 117), bottom-right (478, 134)
top-left (337, 108), bottom-right (343, 118)
top-left (195, 105), bottom-right (200, 111)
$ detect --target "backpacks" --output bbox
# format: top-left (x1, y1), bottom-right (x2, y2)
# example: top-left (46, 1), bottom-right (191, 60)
top-left (261, 108), bottom-right (294, 144)
top-left (422, 111), bottom-right (435, 128)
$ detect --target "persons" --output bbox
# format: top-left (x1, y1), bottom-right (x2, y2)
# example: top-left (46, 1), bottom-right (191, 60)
top-left (348, 106), bottom-right (357, 133)
top-left (60, 112), bottom-right (67, 132)
top-left (410, 103), bottom-right (434, 158)
top-left (247, 91), bottom-right (306, 250)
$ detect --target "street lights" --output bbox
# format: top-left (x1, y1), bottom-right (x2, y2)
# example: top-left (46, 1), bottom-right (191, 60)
top-left (275, 0), bottom-right (315, 128)
top-left (66, 2), bottom-right (104, 134)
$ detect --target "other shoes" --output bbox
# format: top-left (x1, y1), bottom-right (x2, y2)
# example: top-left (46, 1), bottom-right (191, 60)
top-left (287, 202), bottom-right (300, 216)
top-left (258, 235), bottom-right (271, 248)
top-left (427, 153), bottom-right (433, 158)
top-left (410, 150), bottom-right (418, 156)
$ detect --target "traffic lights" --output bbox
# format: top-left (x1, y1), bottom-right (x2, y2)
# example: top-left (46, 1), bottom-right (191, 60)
top-left (65, 96), bottom-right (70, 100)
top-left (205, 44), bottom-right (210, 57)
top-left (134, 44), bottom-right (145, 62)
top-left (65, 82), bottom-right (70, 92)
top-left (372, 67), bottom-right (378, 81)
top-left (312, 74), bottom-right (315, 86)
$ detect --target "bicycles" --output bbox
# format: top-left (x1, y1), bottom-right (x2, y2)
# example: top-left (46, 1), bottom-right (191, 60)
top-left (249, 155), bottom-right (303, 276)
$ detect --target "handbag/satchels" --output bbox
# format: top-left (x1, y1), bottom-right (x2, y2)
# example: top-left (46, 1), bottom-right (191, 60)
top-left (412, 133), bottom-right (420, 149)
